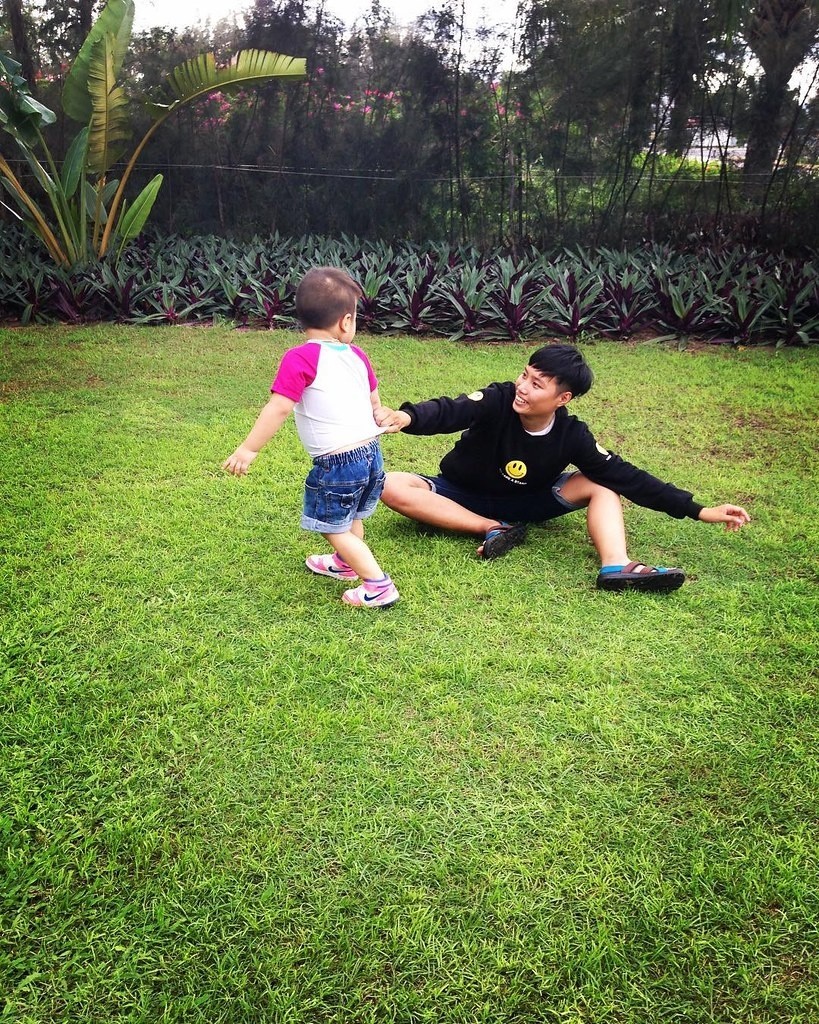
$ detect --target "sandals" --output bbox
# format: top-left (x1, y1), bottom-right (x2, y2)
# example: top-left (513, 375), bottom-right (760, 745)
top-left (479, 523), bottom-right (527, 561)
top-left (597, 561), bottom-right (688, 595)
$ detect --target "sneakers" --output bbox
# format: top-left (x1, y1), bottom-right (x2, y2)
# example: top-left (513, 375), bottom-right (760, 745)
top-left (306, 551), bottom-right (360, 581)
top-left (341, 573), bottom-right (399, 611)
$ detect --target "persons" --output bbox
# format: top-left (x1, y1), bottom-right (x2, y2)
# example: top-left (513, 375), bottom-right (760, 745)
top-left (372, 344), bottom-right (751, 598)
top-left (220, 264), bottom-right (403, 610)
